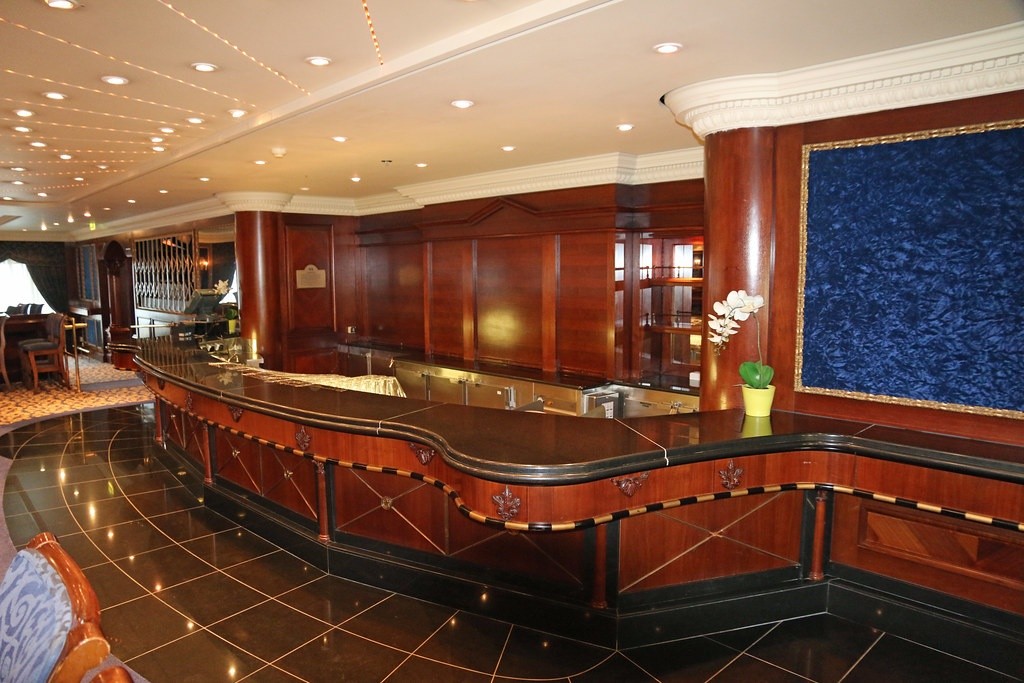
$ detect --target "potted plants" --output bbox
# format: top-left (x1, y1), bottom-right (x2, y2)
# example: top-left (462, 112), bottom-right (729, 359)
top-left (225, 309), bottom-right (236, 334)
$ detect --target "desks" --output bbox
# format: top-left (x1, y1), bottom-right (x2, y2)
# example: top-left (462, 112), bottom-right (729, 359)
top-left (0, 312), bottom-right (64, 384)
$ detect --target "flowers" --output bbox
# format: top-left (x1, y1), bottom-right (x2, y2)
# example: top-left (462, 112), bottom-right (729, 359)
top-left (707, 290), bottom-right (774, 389)
top-left (213, 279), bottom-right (231, 295)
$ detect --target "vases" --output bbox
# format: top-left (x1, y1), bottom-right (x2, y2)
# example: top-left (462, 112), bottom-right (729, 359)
top-left (740, 383), bottom-right (776, 417)
top-left (741, 415), bottom-right (774, 439)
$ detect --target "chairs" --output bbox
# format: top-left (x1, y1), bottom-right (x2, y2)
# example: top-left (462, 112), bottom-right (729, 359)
top-left (5, 306), bottom-right (21, 313)
top-left (0, 532), bottom-right (134, 683)
top-left (17, 302), bottom-right (29, 315)
top-left (21, 313), bottom-right (71, 395)
top-left (16, 313), bottom-right (61, 390)
top-left (30, 304), bottom-right (44, 314)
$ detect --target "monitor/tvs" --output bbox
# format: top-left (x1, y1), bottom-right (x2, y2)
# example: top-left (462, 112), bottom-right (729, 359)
top-left (186, 289), bottom-right (222, 322)
